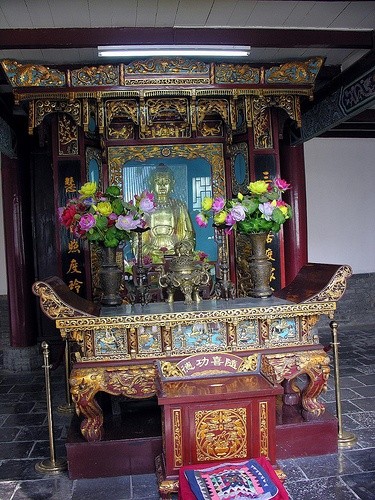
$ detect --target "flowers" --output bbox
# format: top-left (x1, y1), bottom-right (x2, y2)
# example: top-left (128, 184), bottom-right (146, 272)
top-left (57, 181), bottom-right (155, 249)
top-left (195, 176), bottom-right (293, 235)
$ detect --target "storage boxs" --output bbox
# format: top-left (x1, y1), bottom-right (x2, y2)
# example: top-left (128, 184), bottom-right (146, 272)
top-left (154, 371), bottom-right (287, 498)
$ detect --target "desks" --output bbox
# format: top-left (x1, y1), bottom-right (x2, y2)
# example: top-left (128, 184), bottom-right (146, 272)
top-left (31, 262), bottom-right (353, 444)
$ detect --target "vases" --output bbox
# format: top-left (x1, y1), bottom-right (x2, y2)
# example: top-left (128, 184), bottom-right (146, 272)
top-left (100, 247), bottom-right (123, 305)
top-left (247, 233), bottom-right (273, 299)
